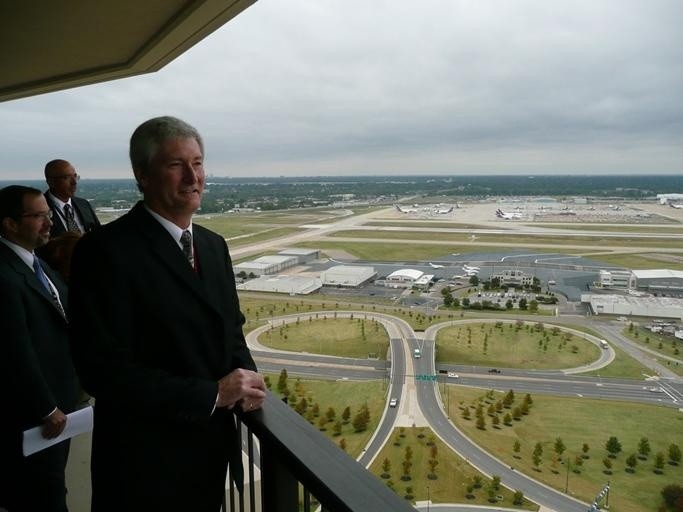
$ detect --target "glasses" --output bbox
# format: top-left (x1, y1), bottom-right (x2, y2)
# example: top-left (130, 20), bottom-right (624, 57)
top-left (22, 210), bottom-right (54, 220)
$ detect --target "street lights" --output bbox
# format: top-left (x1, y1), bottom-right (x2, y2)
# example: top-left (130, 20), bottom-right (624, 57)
top-left (425, 486), bottom-right (429, 511)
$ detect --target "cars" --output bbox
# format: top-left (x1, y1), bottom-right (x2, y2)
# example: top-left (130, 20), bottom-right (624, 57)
top-left (448, 373), bottom-right (458, 379)
top-left (488, 369), bottom-right (500, 373)
top-left (650, 387), bottom-right (663, 392)
top-left (600, 339), bottom-right (608, 349)
top-left (413, 349), bottom-right (421, 359)
top-left (390, 399), bottom-right (396, 407)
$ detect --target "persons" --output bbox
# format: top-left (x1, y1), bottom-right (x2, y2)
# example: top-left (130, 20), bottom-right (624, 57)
top-left (34, 159), bottom-right (98, 285)
top-left (66, 115), bottom-right (265, 510)
top-left (0, 185), bottom-right (83, 512)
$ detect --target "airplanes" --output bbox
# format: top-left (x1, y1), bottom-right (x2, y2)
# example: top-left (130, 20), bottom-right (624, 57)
top-left (428, 262), bottom-right (480, 275)
top-left (393, 203), bottom-right (459, 213)
top-left (496, 209), bottom-right (524, 219)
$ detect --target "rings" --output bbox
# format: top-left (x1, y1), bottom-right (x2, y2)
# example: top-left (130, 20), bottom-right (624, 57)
top-left (250, 403), bottom-right (252, 408)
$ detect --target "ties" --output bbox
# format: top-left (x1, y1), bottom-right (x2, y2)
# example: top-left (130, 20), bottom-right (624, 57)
top-left (178, 230), bottom-right (192, 265)
top-left (64, 204), bottom-right (82, 235)
top-left (33, 254), bottom-right (66, 319)
